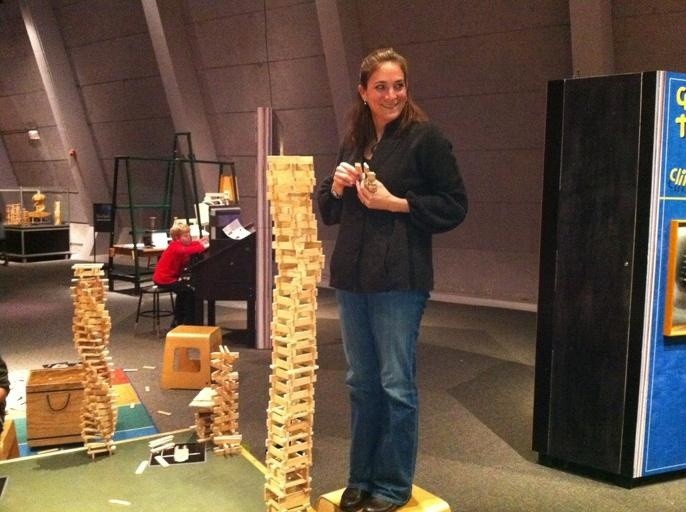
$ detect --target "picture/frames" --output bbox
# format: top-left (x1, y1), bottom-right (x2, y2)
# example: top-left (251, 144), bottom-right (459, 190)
top-left (660, 217), bottom-right (686, 340)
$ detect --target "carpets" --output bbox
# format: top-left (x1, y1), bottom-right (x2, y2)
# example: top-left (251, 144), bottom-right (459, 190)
top-left (4, 367), bottom-right (161, 457)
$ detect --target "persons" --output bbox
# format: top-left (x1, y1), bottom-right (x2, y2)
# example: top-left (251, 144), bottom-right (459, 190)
top-left (318, 46), bottom-right (468, 512)
top-left (153, 223), bottom-right (211, 330)
top-left (0, 357), bottom-right (11, 440)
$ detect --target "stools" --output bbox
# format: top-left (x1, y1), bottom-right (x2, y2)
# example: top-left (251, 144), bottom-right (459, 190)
top-left (0, 416), bottom-right (22, 462)
top-left (314, 474), bottom-right (451, 512)
top-left (187, 386), bottom-right (219, 428)
top-left (134, 283), bottom-right (178, 346)
top-left (160, 325), bottom-right (228, 390)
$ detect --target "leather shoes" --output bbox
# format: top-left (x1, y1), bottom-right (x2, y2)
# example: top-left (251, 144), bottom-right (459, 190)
top-left (361, 482), bottom-right (411, 512)
top-left (336, 482), bottom-right (371, 512)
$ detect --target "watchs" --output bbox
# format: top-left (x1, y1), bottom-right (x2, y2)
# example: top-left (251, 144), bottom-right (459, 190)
top-left (330, 189), bottom-right (342, 200)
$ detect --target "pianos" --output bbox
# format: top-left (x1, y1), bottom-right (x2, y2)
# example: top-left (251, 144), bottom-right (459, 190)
top-left (177, 224), bottom-right (255, 350)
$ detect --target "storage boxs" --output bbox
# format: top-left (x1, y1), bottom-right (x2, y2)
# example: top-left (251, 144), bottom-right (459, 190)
top-left (22, 365), bottom-right (93, 450)
